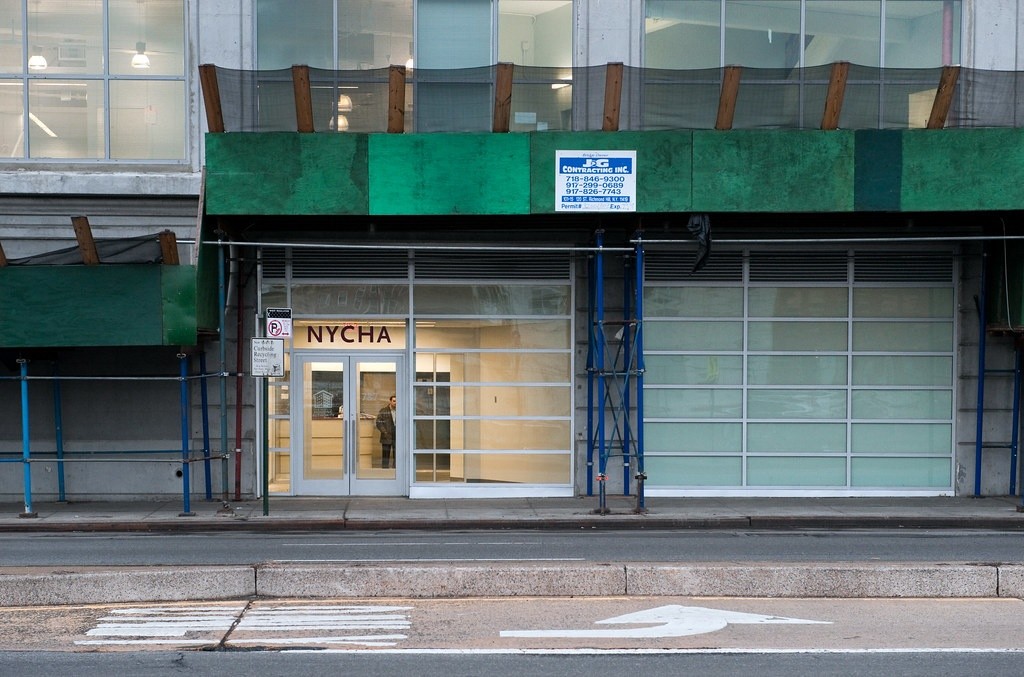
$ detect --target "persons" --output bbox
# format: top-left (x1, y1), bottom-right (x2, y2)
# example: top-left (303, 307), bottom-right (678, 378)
top-left (375, 395), bottom-right (397, 469)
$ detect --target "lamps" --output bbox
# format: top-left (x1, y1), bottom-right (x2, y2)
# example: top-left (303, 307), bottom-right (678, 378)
top-left (406, 42), bottom-right (414, 71)
top-left (329, 115), bottom-right (349, 131)
top-left (332, 93), bottom-right (353, 111)
top-left (131, 42), bottom-right (151, 68)
top-left (28, 45), bottom-right (49, 69)
